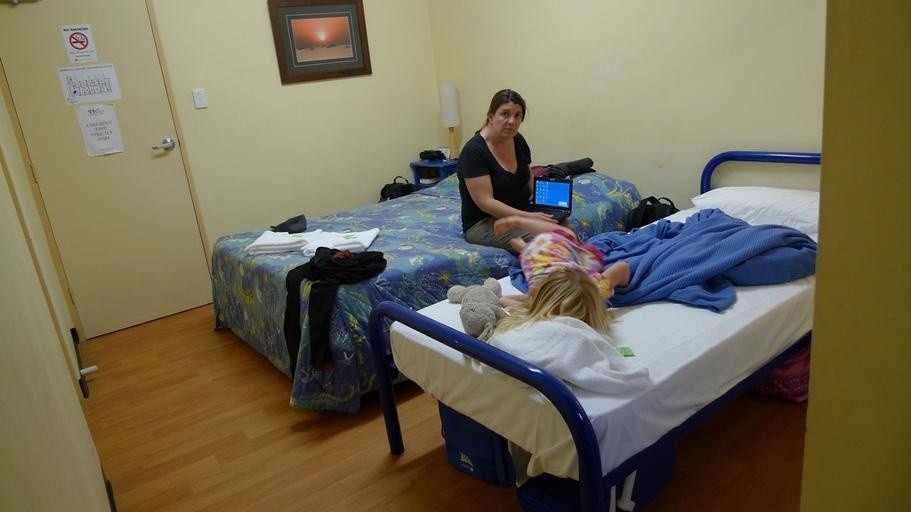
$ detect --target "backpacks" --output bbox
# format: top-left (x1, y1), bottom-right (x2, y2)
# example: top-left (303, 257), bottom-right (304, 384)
top-left (377, 175), bottom-right (419, 203)
top-left (626, 195), bottom-right (681, 232)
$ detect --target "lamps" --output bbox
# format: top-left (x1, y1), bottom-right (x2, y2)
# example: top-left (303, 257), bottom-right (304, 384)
top-left (440, 82), bottom-right (460, 159)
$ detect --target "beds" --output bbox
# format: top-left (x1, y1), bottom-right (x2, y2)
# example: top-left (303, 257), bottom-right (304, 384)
top-left (209, 150), bottom-right (639, 411)
top-left (371, 150), bottom-right (822, 511)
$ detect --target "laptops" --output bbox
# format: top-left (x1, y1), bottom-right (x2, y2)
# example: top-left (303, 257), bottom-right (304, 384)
top-left (524, 176), bottom-right (572, 223)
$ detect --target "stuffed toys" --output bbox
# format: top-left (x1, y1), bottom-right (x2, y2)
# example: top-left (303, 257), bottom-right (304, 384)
top-left (447, 275), bottom-right (506, 361)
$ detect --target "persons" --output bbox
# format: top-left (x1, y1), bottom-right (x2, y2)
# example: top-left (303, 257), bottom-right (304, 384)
top-left (490, 213), bottom-right (633, 337)
top-left (455, 87), bottom-right (560, 257)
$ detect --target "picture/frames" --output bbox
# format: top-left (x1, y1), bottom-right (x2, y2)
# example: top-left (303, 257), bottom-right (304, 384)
top-left (267, 0), bottom-right (372, 86)
